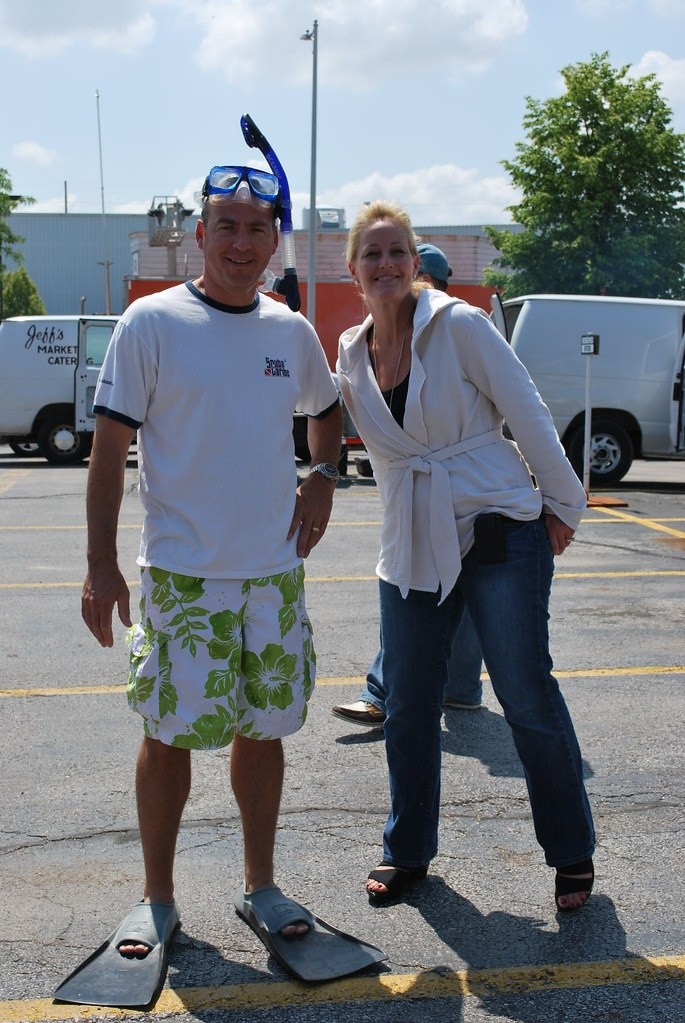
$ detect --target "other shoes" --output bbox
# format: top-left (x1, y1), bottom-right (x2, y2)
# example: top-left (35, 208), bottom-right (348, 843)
top-left (443, 695), bottom-right (482, 709)
top-left (330, 702), bottom-right (386, 727)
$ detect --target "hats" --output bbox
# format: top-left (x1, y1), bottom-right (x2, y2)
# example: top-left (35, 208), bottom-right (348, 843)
top-left (417, 242), bottom-right (452, 287)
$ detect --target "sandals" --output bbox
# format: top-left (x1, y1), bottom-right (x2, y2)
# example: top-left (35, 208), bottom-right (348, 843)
top-left (365, 857), bottom-right (431, 899)
top-left (555, 856), bottom-right (594, 911)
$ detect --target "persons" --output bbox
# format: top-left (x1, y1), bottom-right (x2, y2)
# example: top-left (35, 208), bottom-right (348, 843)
top-left (336, 202), bottom-right (599, 914)
top-left (84, 165), bottom-right (344, 954)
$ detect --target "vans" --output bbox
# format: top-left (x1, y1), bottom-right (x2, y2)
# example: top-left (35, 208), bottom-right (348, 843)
top-left (488, 293), bottom-right (685, 490)
top-left (0, 316), bottom-right (136, 468)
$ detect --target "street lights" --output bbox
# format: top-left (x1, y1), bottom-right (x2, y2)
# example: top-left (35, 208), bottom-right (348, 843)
top-left (298, 18), bottom-right (321, 333)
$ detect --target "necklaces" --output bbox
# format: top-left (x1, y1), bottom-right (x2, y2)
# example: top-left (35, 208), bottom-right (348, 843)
top-left (373, 305), bottom-right (415, 412)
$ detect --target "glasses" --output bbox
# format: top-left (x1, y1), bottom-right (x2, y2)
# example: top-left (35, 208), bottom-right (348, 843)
top-left (203, 166), bottom-right (281, 202)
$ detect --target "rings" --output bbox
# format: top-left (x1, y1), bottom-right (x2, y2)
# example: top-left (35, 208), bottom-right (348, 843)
top-left (312, 527), bottom-right (319, 532)
top-left (565, 539), bottom-right (570, 544)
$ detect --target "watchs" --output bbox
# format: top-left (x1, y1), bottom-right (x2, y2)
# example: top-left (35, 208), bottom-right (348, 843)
top-left (309, 462), bottom-right (340, 485)
